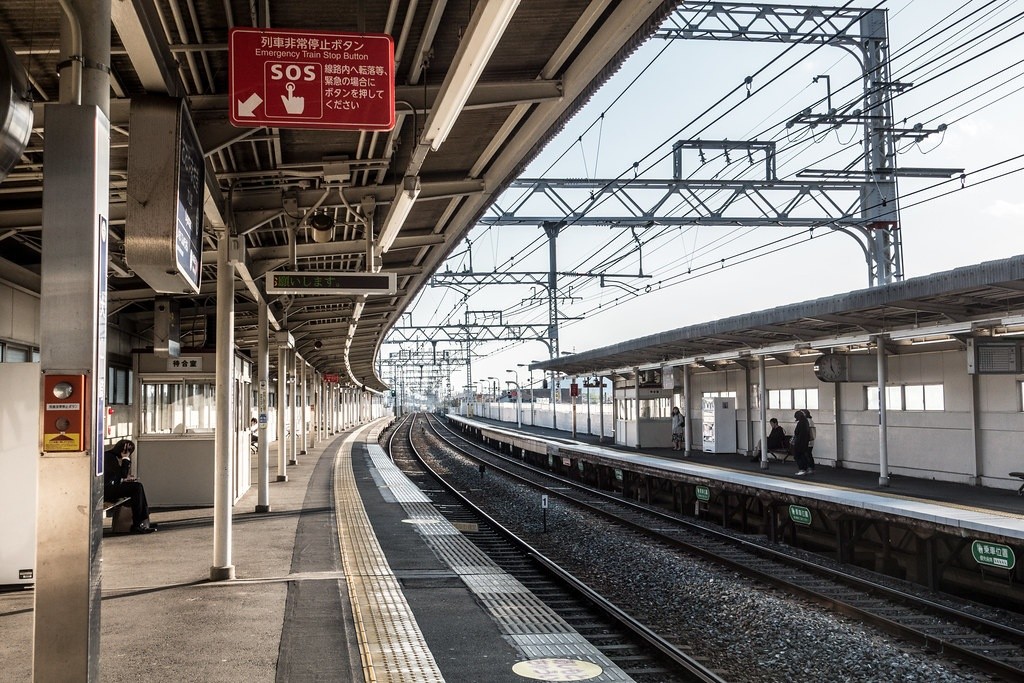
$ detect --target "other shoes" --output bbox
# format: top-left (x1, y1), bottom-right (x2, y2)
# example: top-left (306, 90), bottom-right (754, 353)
top-left (672, 448), bottom-right (677, 451)
top-left (676, 448), bottom-right (681, 451)
top-left (750, 453), bottom-right (762, 463)
top-left (807, 467), bottom-right (816, 475)
top-left (130, 521), bottom-right (157, 534)
top-left (795, 470), bottom-right (807, 476)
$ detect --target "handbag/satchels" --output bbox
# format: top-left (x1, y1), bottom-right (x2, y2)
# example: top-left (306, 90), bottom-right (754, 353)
top-left (808, 427), bottom-right (816, 441)
top-left (789, 435), bottom-right (796, 445)
top-left (678, 414), bottom-right (685, 427)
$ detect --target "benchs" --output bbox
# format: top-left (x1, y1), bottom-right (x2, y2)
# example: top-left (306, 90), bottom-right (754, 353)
top-left (250, 436), bottom-right (258, 454)
top-left (103, 471), bottom-right (136, 514)
top-left (286, 424), bottom-right (300, 438)
top-left (767, 435), bottom-right (794, 463)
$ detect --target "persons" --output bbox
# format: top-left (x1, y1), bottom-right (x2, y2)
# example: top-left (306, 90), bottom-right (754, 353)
top-left (671, 407), bottom-right (684, 450)
top-left (791, 411), bottom-right (810, 476)
top-left (750, 418), bottom-right (785, 462)
top-left (251, 417), bottom-right (258, 443)
top-left (799, 409), bottom-right (817, 474)
top-left (104, 439), bottom-right (157, 533)
